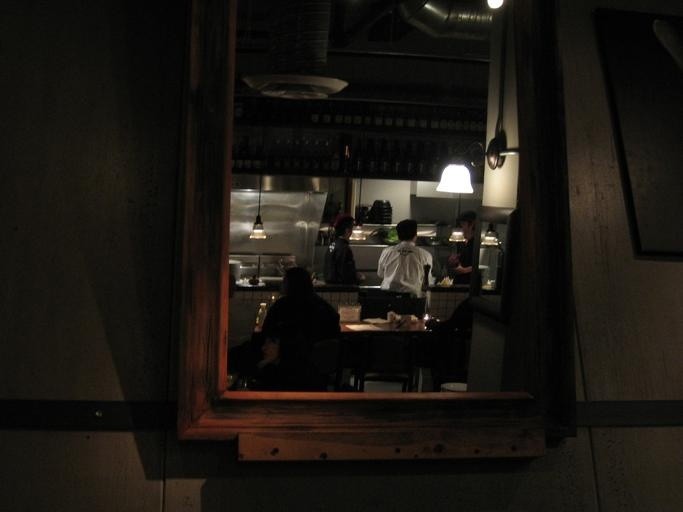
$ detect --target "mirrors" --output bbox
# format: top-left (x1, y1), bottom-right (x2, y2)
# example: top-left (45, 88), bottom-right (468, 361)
top-left (174, 0), bottom-right (553, 460)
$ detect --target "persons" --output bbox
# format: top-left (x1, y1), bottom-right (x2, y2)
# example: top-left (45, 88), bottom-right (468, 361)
top-left (376, 218), bottom-right (434, 310)
top-left (323, 212), bottom-right (366, 286)
top-left (435, 212), bottom-right (478, 288)
top-left (239, 268), bottom-right (340, 391)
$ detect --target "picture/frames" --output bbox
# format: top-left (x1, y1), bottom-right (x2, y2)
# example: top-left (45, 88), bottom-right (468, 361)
top-left (587, 0), bottom-right (683, 261)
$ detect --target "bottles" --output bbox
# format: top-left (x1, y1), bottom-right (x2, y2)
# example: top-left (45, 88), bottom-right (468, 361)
top-left (256, 303), bottom-right (267, 325)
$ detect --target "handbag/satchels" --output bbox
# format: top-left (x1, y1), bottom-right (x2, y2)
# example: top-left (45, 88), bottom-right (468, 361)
top-left (422, 265), bottom-right (431, 290)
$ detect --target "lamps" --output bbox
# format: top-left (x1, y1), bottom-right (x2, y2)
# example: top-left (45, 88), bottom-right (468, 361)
top-left (348, 179), bottom-right (367, 240)
top-left (249, 176), bottom-right (267, 238)
top-left (436, 137), bottom-right (500, 194)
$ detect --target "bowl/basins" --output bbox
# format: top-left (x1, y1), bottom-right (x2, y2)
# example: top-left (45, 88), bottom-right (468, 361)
top-left (369, 199), bottom-right (393, 224)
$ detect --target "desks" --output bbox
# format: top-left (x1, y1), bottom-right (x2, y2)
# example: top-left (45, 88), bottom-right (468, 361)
top-left (253, 318), bottom-right (442, 392)
